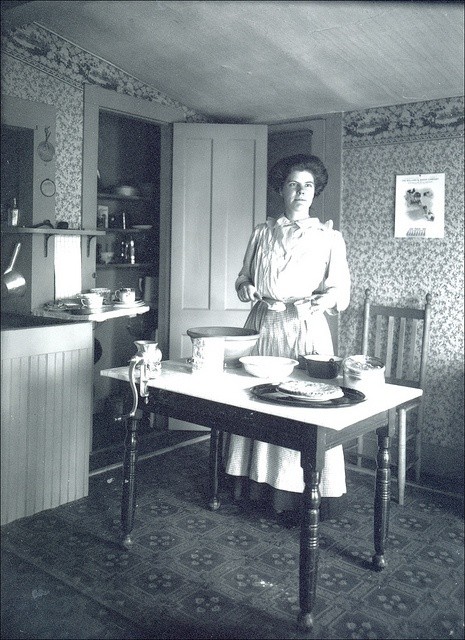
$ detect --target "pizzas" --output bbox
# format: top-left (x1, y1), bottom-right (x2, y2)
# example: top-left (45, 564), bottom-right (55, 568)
top-left (277, 380), bottom-right (343, 398)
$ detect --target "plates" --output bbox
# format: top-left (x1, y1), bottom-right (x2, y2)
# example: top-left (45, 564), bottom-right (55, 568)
top-left (131, 224), bottom-right (153, 229)
top-left (112, 300), bottom-right (146, 309)
top-left (247, 382), bottom-right (367, 408)
top-left (275, 388), bottom-right (345, 401)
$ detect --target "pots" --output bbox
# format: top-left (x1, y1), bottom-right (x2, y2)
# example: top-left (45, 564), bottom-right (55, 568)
top-left (106, 182), bottom-right (140, 197)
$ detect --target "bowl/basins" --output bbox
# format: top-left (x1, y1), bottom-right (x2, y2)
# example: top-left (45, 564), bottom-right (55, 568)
top-left (303, 354), bottom-right (343, 379)
top-left (89, 288), bottom-right (111, 299)
top-left (238, 355), bottom-right (299, 378)
top-left (341, 355), bottom-right (386, 392)
top-left (186, 326), bottom-right (262, 369)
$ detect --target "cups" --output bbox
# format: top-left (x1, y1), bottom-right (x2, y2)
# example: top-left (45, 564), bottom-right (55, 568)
top-left (81, 296), bottom-right (104, 308)
top-left (293, 299), bottom-right (312, 320)
top-left (114, 287), bottom-right (135, 303)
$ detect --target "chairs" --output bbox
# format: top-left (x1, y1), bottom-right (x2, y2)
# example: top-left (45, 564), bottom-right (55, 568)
top-left (342, 289), bottom-right (433, 506)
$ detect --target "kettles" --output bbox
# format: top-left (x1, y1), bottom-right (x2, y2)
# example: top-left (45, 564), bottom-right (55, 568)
top-left (138, 274), bottom-right (158, 303)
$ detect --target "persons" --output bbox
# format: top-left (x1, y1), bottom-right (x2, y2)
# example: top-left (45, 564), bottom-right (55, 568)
top-left (219, 154), bottom-right (351, 514)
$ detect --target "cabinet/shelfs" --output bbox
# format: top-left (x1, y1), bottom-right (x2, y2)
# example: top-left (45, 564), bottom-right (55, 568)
top-left (98, 193), bottom-right (160, 270)
top-left (0, 225), bottom-right (150, 321)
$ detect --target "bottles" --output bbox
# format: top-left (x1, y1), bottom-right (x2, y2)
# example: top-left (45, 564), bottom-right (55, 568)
top-left (6, 198), bottom-right (21, 227)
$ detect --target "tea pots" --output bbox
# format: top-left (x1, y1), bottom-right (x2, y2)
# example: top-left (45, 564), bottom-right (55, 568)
top-left (131, 340), bottom-right (163, 369)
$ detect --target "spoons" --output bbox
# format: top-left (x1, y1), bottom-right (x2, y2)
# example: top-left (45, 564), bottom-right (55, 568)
top-left (255, 297), bottom-right (286, 312)
